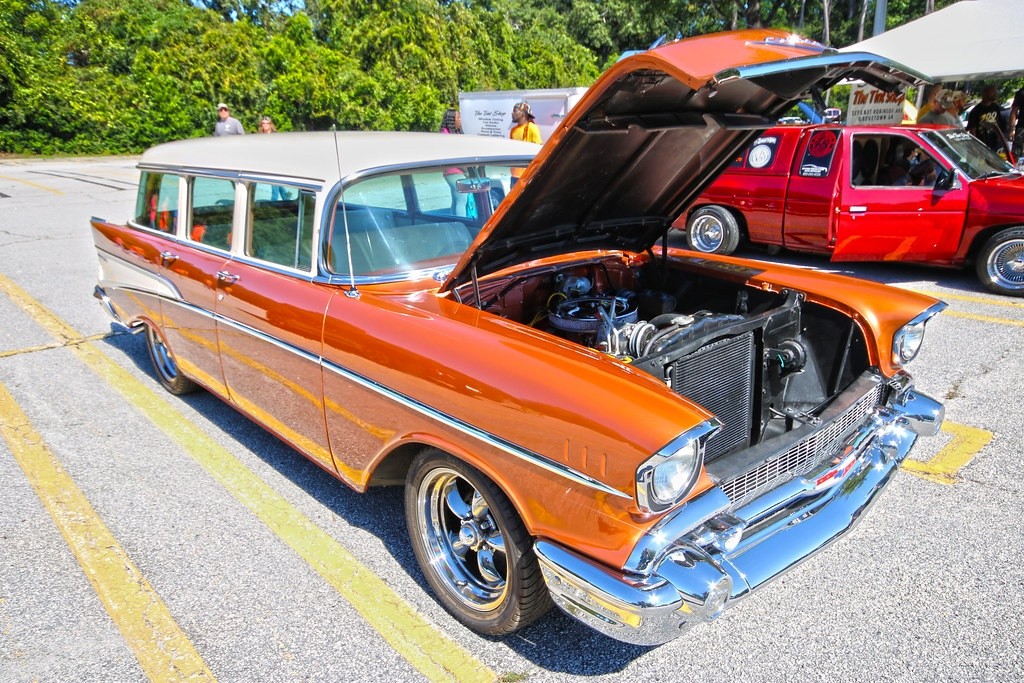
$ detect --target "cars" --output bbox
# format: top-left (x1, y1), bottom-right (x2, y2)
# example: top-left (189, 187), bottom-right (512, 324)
top-left (670, 123), bottom-right (1024, 298)
top-left (89, 29), bottom-right (949, 649)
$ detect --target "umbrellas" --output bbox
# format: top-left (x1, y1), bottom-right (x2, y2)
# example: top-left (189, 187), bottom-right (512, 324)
top-left (835, 0), bottom-right (1024, 82)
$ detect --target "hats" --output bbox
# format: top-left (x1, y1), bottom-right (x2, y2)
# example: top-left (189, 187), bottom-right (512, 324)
top-left (514, 102), bottom-right (535, 119)
top-left (217, 103), bottom-right (228, 111)
top-left (936, 89), bottom-right (956, 109)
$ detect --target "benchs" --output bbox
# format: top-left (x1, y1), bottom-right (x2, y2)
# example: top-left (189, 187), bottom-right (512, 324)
top-left (189, 209), bottom-right (393, 257)
top-left (268, 221), bottom-right (476, 276)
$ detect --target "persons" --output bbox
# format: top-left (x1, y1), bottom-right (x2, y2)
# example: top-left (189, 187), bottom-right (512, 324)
top-left (510, 102), bottom-right (542, 189)
top-left (259, 117), bottom-right (290, 201)
top-left (215, 102), bottom-right (245, 189)
top-left (440, 109), bottom-right (469, 217)
top-left (897, 84), bottom-right (1024, 187)
